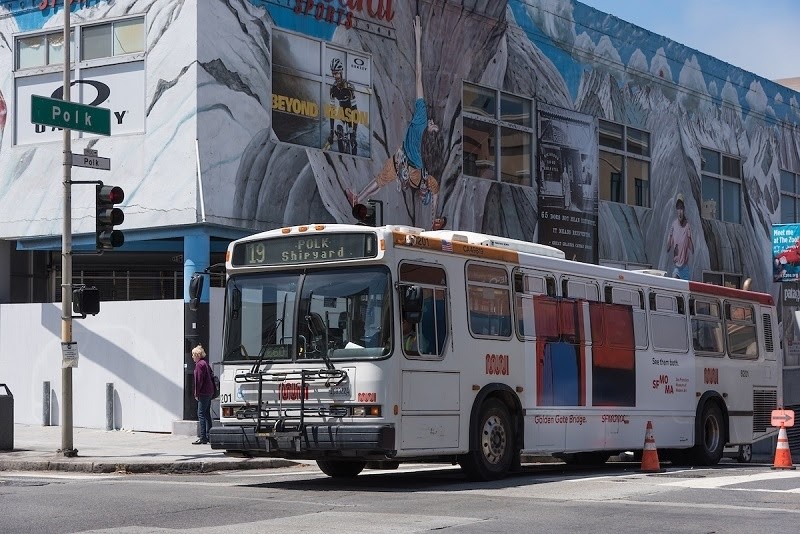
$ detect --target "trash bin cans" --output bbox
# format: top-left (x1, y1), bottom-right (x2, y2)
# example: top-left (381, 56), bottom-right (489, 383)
top-left (0, 383), bottom-right (14, 451)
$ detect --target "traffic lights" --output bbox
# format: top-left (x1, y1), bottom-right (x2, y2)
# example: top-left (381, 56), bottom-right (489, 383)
top-left (72, 287), bottom-right (100, 314)
top-left (95, 184), bottom-right (124, 251)
top-left (352, 202), bottom-right (377, 227)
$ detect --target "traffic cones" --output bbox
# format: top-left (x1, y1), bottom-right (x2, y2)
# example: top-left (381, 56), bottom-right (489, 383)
top-left (770, 426), bottom-right (796, 470)
top-left (633, 420), bottom-right (667, 473)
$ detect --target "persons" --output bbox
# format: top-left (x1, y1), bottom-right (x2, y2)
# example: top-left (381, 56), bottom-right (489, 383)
top-left (191, 345), bottom-right (214, 444)
top-left (381, 314), bottom-right (424, 353)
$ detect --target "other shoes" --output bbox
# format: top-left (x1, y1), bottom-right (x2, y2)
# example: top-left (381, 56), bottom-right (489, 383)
top-left (192, 438), bottom-right (207, 444)
top-left (207, 438), bottom-right (212, 443)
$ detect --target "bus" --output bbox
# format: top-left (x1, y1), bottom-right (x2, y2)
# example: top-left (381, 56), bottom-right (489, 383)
top-left (206, 220), bottom-right (786, 483)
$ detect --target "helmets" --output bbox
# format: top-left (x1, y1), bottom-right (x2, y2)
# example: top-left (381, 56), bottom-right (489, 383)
top-left (330, 58), bottom-right (343, 71)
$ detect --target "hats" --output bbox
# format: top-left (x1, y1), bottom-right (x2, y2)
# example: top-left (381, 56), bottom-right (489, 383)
top-left (676, 193), bottom-right (686, 205)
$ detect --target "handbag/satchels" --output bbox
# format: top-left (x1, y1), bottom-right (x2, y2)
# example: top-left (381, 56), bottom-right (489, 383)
top-left (212, 376), bottom-right (221, 398)
